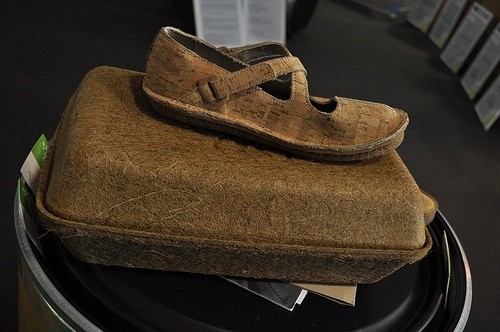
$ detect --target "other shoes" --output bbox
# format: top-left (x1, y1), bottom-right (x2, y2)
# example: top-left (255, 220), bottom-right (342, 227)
top-left (141, 25), bottom-right (409, 163)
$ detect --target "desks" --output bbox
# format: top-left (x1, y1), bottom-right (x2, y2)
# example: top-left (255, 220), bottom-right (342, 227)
top-left (14, 153), bottom-right (472, 332)
